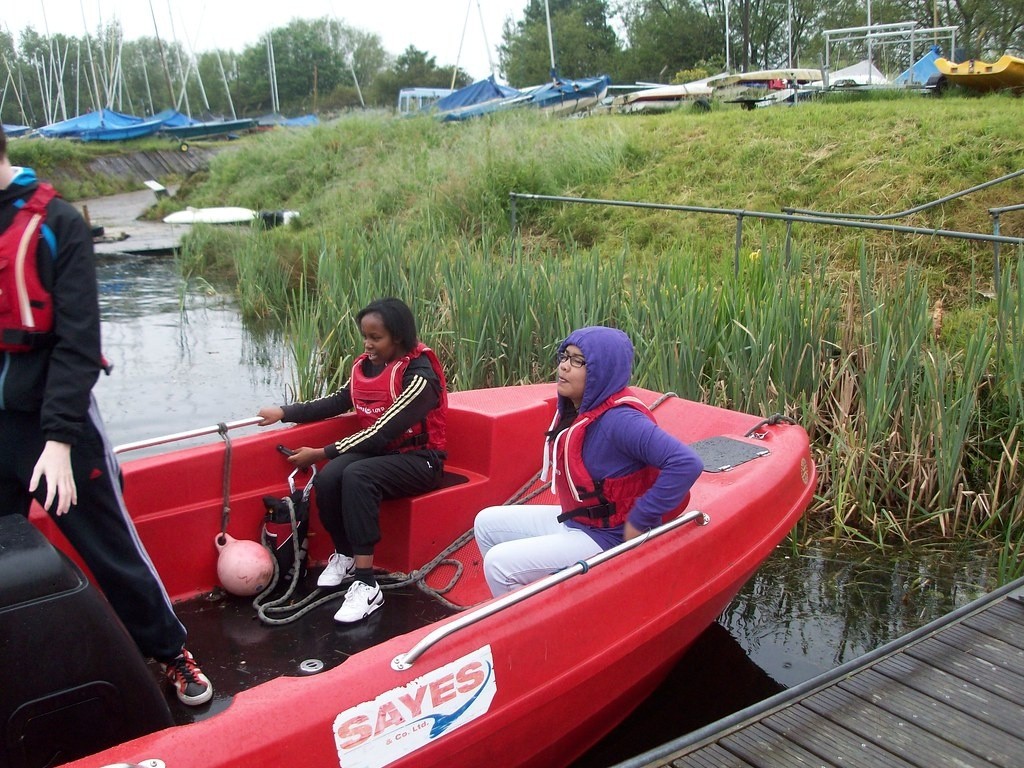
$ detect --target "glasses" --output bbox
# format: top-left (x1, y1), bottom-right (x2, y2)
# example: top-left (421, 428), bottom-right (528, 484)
top-left (558, 352), bottom-right (586, 368)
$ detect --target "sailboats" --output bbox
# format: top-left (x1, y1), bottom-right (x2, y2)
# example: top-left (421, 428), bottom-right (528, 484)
top-left (398, 0), bottom-right (945, 130)
top-left (0, 0), bottom-right (318, 141)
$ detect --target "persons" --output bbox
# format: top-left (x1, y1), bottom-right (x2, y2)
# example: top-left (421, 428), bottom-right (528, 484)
top-left (473, 324), bottom-right (704, 598)
top-left (0, 123), bottom-right (214, 706)
top-left (257, 298), bottom-right (449, 624)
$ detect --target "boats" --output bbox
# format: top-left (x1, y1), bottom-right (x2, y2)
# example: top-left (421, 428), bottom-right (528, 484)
top-left (933, 55), bottom-right (1024, 96)
top-left (161, 205), bottom-right (260, 224)
top-left (0, 384), bottom-right (819, 768)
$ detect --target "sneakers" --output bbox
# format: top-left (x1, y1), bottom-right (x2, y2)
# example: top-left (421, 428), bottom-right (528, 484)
top-left (333, 581), bottom-right (385, 623)
top-left (159, 647), bottom-right (213, 705)
top-left (317, 549), bottom-right (357, 586)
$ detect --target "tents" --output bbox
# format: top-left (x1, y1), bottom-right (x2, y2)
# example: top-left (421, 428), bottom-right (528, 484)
top-left (1, 109), bottom-right (238, 143)
top-left (419, 78), bottom-right (521, 115)
top-left (281, 114), bottom-right (319, 126)
